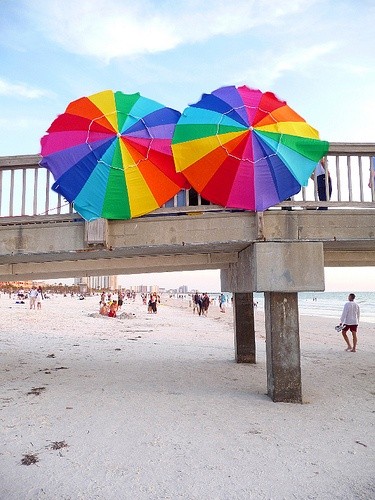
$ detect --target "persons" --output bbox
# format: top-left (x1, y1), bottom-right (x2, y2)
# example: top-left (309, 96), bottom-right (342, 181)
top-left (218, 294), bottom-right (233, 312)
top-left (16, 288), bottom-right (28, 301)
top-left (140, 292), bottom-right (161, 314)
top-left (36, 293), bottom-right (42, 309)
top-left (99, 289), bottom-right (137, 318)
top-left (37, 286), bottom-right (43, 295)
top-left (311, 155), bottom-right (334, 210)
top-left (281, 198), bottom-right (294, 210)
top-left (339, 293), bottom-right (360, 352)
top-left (367, 157), bottom-right (375, 203)
top-left (29, 286), bottom-right (38, 310)
top-left (192, 291), bottom-right (218, 317)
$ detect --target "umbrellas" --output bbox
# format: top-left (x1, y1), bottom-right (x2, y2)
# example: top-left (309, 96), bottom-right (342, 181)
top-left (169, 84), bottom-right (329, 213)
top-left (38, 89), bottom-right (192, 224)
